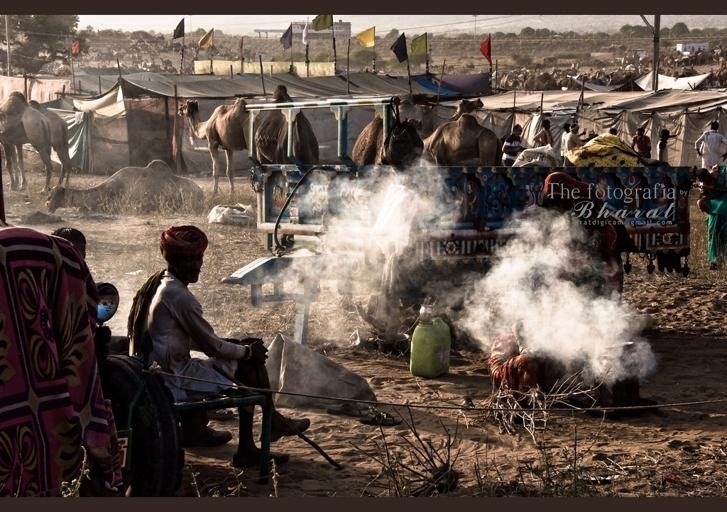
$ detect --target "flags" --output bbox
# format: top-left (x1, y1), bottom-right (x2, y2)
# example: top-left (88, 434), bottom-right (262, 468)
top-left (478, 37), bottom-right (491, 60)
top-left (171, 18), bottom-right (185, 39)
top-left (312, 15), bottom-right (332, 31)
top-left (279, 25), bottom-right (292, 51)
top-left (389, 31), bottom-right (408, 62)
top-left (200, 27), bottom-right (214, 49)
top-left (411, 33), bottom-right (427, 54)
top-left (356, 26), bottom-right (374, 48)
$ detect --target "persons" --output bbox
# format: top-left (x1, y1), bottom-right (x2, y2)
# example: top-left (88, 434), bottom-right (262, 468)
top-left (497, 116), bottom-right (727, 169)
top-left (697, 153), bottom-right (727, 271)
top-left (126, 223), bottom-right (311, 468)
top-left (52, 224), bottom-right (232, 449)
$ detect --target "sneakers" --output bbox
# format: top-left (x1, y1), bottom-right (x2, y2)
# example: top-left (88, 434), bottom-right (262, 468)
top-left (260, 411), bottom-right (310, 442)
top-left (207, 408), bottom-right (233, 420)
top-left (233, 442), bottom-right (289, 467)
top-left (179, 426), bottom-right (232, 448)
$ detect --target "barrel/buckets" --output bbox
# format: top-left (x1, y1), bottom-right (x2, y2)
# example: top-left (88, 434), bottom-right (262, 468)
top-left (410, 317), bottom-right (451, 377)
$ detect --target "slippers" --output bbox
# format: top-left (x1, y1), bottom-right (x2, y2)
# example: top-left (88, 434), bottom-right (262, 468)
top-left (360, 411), bottom-right (402, 426)
top-left (327, 403), bottom-right (371, 417)
